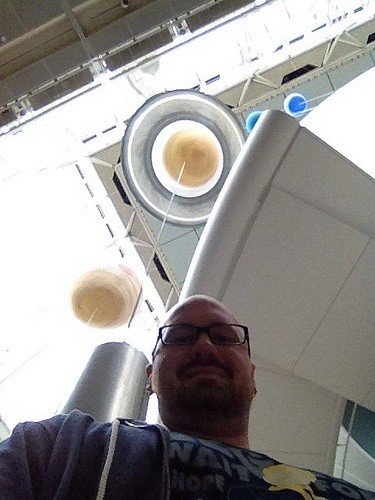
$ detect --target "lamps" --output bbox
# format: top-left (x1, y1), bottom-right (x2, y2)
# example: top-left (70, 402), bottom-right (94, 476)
top-left (57, 61), bottom-right (375, 426)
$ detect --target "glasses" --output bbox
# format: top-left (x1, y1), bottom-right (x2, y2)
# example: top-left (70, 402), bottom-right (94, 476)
top-left (151, 322), bottom-right (251, 361)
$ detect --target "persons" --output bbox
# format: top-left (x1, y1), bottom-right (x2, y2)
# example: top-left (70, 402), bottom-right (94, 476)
top-left (0, 294), bottom-right (375, 500)
